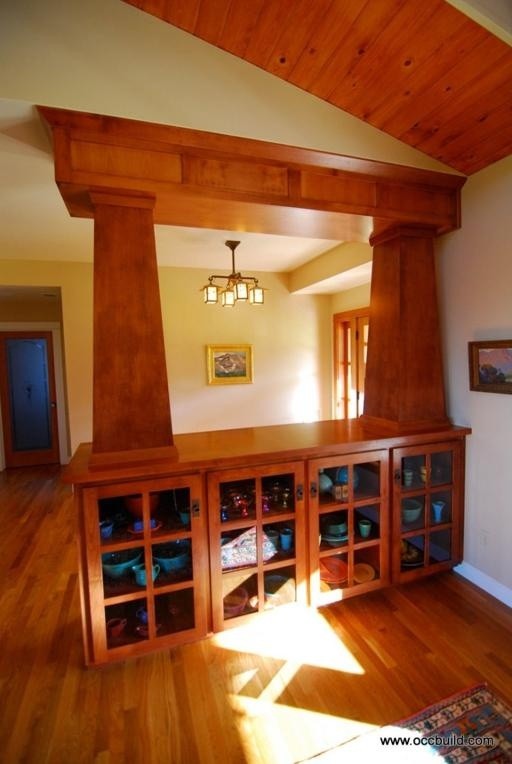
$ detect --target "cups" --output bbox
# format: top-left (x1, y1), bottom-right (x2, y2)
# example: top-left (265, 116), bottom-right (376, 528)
top-left (266, 527), bottom-right (294, 552)
top-left (403, 466), bottom-right (432, 487)
top-left (180, 507), bottom-right (190, 525)
top-left (106, 617), bottom-right (128, 637)
top-left (132, 562), bottom-right (163, 588)
top-left (359, 520), bottom-right (372, 538)
top-left (99, 520), bottom-right (113, 539)
top-left (433, 501), bottom-right (446, 523)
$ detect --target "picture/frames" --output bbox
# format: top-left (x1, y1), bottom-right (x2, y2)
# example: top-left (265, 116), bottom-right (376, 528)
top-left (206, 343), bottom-right (252, 385)
top-left (468, 337), bottom-right (512, 395)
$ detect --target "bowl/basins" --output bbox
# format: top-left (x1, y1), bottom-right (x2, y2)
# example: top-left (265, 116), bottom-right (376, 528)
top-left (99, 538), bottom-right (191, 580)
top-left (125, 492), bottom-right (160, 519)
top-left (404, 499), bottom-right (422, 524)
top-left (224, 576), bottom-right (290, 613)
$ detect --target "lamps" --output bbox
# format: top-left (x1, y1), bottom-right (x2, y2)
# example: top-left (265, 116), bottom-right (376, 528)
top-left (200, 239), bottom-right (268, 308)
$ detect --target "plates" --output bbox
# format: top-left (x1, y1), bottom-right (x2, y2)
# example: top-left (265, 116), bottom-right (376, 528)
top-left (127, 521), bottom-right (162, 533)
top-left (319, 558), bottom-right (375, 584)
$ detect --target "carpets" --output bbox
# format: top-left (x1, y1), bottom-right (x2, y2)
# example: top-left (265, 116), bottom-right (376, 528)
top-left (295, 680), bottom-right (512, 760)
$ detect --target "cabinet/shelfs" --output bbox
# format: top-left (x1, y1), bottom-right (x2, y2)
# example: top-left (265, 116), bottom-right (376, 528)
top-left (212, 438), bottom-right (387, 628)
top-left (389, 428), bottom-right (469, 594)
top-left (73, 463), bottom-right (210, 665)
